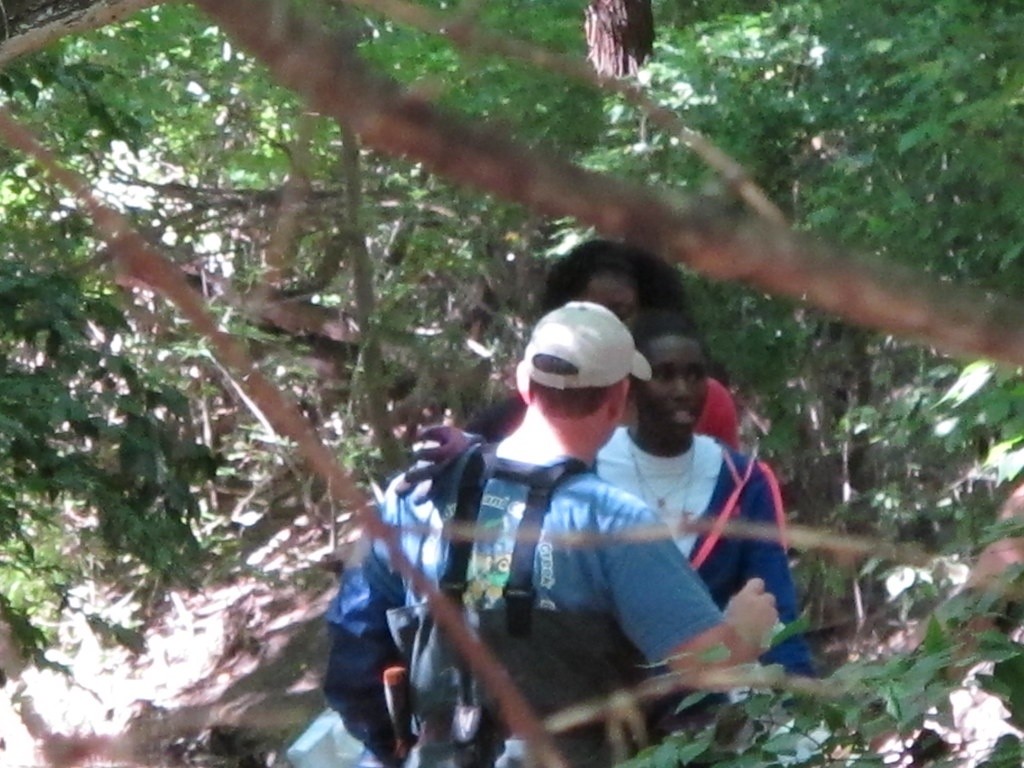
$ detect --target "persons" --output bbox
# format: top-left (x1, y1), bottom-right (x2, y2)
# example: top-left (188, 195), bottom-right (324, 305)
top-left (290, 240), bottom-right (818, 768)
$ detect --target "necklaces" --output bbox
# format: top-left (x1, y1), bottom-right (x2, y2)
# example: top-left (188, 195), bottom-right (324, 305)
top-left (632, 455), bottom-right (692, 508)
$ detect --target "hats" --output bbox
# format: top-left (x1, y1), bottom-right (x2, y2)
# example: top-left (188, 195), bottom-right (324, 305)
top-left (523, 299), bottom-right (653, 390)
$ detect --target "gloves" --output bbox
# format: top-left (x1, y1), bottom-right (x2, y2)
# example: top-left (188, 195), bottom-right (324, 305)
top-left (393, 425), bottom-right (490, 505)
